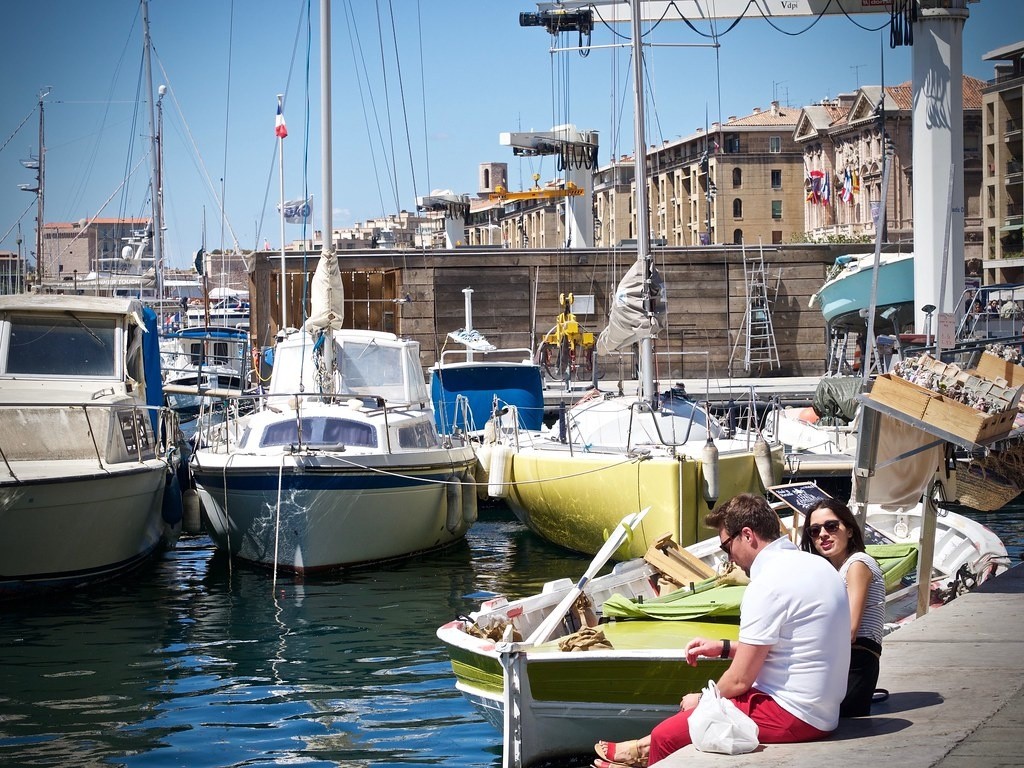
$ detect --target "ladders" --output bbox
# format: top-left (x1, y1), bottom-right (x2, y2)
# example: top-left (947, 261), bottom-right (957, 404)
top-left (726, 262), bottom-right (783, 374)
top-left (740, 234), bottom-right (782, 373)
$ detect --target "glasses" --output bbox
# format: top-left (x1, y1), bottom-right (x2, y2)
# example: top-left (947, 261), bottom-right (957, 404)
top-left (806, 519), bottom-right (840, 538)
top-left (720, 531), bottom-right (740, 553)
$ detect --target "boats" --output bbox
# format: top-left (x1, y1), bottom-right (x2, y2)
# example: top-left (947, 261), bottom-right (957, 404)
top-left (0, 0), bottom-right (479, 588)
top-left (431, 0), bottom-right (1024, 560)
top-left (436, 503), bottom-right (1011, 768)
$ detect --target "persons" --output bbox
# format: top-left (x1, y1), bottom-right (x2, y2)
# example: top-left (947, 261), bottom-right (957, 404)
top-left (648, 493), bottom-right (851, 766)
top-left (589, 499), bottom-right (886, 768)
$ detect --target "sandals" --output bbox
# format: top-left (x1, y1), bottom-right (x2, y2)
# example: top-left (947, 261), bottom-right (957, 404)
top-left (590, 740), bottom-right (648, 768)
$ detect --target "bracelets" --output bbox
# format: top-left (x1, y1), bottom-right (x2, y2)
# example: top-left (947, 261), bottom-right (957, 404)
top-left (720, 639), bottom-right (730, 659)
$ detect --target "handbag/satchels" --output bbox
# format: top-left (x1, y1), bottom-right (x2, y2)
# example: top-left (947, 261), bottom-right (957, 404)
top-left (687, 679), bottom-right (760, 755)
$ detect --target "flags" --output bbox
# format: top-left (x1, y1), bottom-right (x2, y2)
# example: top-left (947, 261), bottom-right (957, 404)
top-left (703, 179), bottom-right (716, 203)
top-left (713, 141), bottom-right (719, 154)
top-left (165, 313), bottom-right (180, 324)
top-left (841, 164), bottom-right (859, 202)
top-left (805, 171), bottom-right (831, 206)
top-left (697, 151), bottom-right (707, 173)
top-left (275, 102), bottom-right (288, 139)
top-left (275, 197), bottom-right (312, 225)
